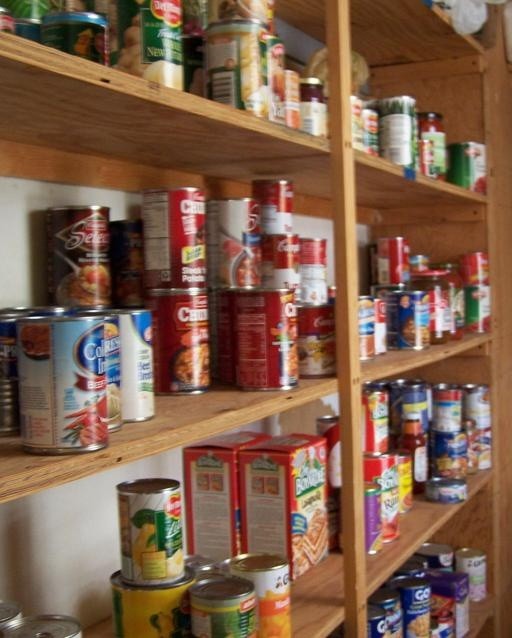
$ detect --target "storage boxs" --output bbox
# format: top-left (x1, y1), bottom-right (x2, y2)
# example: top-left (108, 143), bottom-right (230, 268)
top-left (179, 430), bottom-right (271, 564)
top-left (235, 431), bottom-right (333, 583)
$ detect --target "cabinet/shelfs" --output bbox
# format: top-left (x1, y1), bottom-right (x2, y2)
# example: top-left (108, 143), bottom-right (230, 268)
top-left (344, 0), bottom-right (512, 638)
top-left (0, 1), bottom-right (363, 638)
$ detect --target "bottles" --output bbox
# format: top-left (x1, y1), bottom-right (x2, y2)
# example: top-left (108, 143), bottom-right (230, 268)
top-left (461, 418), bottom-right (480, 475)
top-left (399, 411), bottom-right (426, 493)
top-left (407, 269), bottom-right (453, 343)
top-left (299, 78), bottom-right (325, 137)
top-left (416, 110), bottom-right (447, 181)
top-left (429, 262), bottom-right (466, 340)
